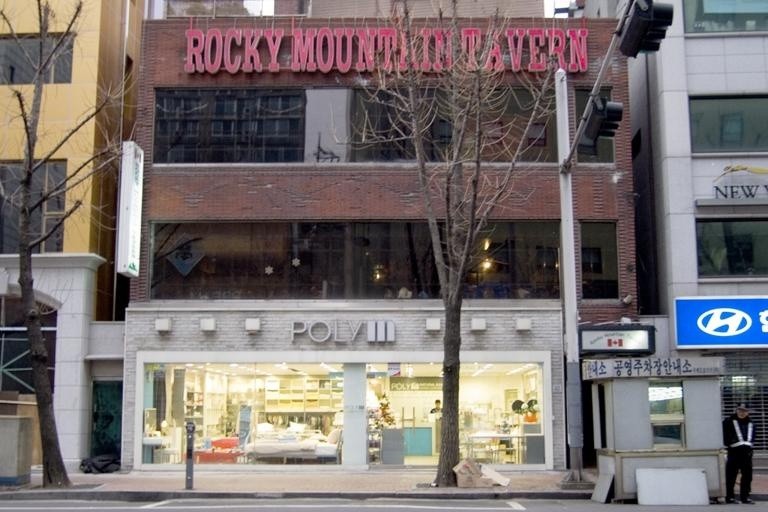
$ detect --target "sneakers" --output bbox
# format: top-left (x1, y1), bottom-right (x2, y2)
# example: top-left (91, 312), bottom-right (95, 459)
top-left (726, 497), bottom-right (739, 503)
top-left (742, 498), bottom-right (756, 504)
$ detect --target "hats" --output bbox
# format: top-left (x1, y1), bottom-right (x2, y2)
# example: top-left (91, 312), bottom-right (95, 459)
top-left (737, 402), bottom-right (749, 411)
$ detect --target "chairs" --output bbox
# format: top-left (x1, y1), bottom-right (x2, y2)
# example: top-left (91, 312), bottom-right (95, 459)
top-left (459, 432), bottom-right (516, 464)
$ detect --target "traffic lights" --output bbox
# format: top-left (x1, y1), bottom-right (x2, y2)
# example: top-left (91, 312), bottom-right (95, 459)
top-left (615, 1), bottom-right (674, 61)
top-left (584, 96), bottom-right (624, 141)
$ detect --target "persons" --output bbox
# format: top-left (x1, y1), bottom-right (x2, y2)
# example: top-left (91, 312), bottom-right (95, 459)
top-left (430, 399), bottom-right (444, 414)
top-left (722, 402), bottom-right (757, 504)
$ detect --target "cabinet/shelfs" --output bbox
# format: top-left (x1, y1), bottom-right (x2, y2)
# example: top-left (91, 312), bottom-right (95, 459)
top-left (229, 376), bottom-right (344, 412)
top-left (174, 369), bottom-right (227, 450)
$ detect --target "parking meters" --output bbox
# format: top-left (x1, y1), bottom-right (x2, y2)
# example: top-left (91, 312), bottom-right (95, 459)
top-left (185, 421), bottom-right (196, 489)
